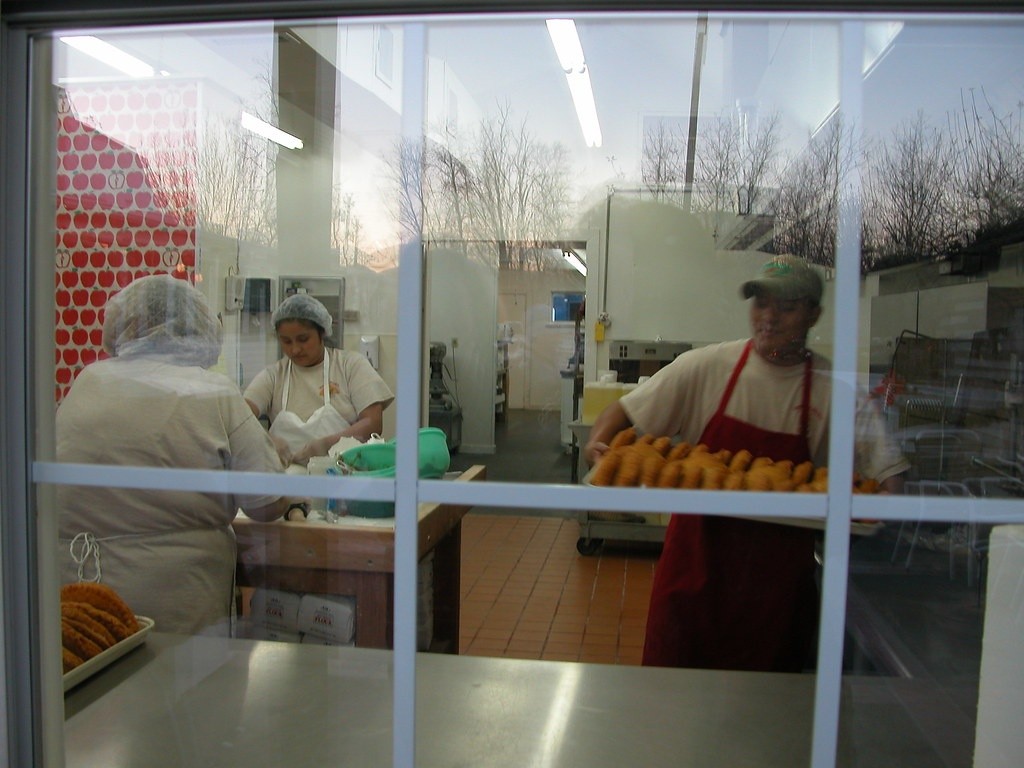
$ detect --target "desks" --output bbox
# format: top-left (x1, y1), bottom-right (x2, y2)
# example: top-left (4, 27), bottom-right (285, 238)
top-left (40, 532), bottom-right (990, 768)
top-left (227, 446), bottom-right (485, 653)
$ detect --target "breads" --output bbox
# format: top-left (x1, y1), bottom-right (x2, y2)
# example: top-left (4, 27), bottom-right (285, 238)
top-left (587, 428), bottom-right (880, 525)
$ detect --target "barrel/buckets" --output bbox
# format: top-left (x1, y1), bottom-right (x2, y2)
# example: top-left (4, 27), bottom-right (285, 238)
top-left (334, 443), bottom-right (397, 519)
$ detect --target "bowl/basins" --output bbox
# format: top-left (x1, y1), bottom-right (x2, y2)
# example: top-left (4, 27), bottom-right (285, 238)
top-left (388, 426), bottom-right (450, 479)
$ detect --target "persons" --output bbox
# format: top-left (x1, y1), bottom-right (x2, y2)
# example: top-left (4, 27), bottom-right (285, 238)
top-left (585, 255), bottom-right (912, 676)
top-left (53, 274), bottom-right (310, 641)
top-left (242, 295), bottom-right (395, 469)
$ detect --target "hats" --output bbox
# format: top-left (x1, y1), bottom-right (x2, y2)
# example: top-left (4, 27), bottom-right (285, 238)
top-left (739, 253), bottom-right (823, 304)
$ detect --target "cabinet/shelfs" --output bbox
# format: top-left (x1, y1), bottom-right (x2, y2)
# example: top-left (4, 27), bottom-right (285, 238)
top-left (494, 339), bottom-right (509, 426)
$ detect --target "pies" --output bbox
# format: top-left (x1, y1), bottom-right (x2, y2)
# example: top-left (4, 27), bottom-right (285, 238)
top-left (60, 580), bottom-right (137, 675)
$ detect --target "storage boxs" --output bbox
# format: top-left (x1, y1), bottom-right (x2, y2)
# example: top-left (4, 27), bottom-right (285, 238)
top-left (361, 335), bottom-right (378, 372)
